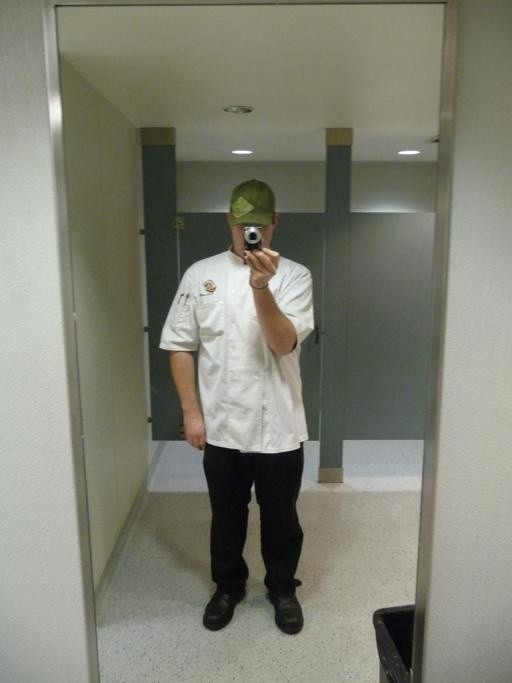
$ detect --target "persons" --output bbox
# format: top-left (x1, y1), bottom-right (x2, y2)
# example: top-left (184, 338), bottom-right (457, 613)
top-left (156, 175), bottom-right (321, 638)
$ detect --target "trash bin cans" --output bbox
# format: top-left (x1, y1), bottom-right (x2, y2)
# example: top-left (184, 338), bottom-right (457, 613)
top-left (372, 605), bottom-right (415, 683)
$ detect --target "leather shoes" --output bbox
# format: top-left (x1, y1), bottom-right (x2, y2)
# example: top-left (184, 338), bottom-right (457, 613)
top-left (203, 586), bottom-right (247, 632)
top-left (265, 588), bottom-right (304, 635)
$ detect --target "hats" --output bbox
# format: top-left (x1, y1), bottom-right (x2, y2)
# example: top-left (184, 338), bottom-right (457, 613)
top-left (228, 178), bottom-right (275, 227)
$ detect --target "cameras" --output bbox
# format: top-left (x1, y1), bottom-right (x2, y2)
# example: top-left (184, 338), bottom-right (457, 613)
top-left (243, 227), bottom-right (264, 265)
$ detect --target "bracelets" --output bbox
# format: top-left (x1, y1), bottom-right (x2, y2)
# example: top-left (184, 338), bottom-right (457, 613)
top-left (248, 278), bottom-right (269, 291)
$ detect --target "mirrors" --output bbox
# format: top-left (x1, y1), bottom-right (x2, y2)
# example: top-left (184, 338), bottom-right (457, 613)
top-left (41, 2), bottom-right (461, 683)
